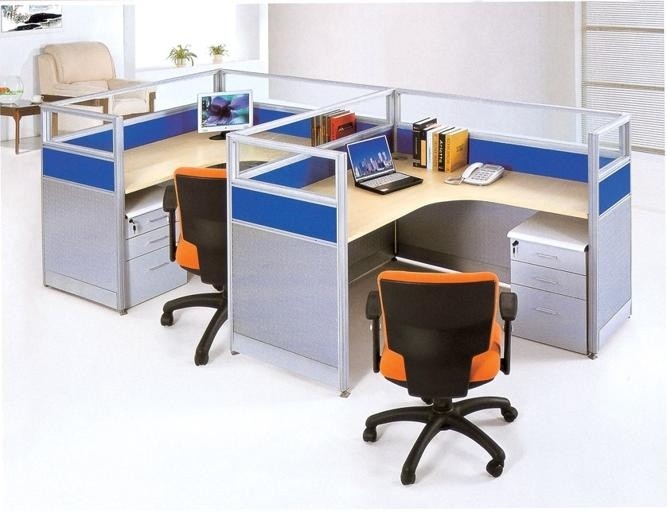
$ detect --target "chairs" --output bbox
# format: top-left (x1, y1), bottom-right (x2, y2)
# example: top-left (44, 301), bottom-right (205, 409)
top-left (361, 270), bottom-right (518, 484)
top-left (38, 41), bottom-right (156, 139)
top-left (160, 166), bottom-right (227, 366)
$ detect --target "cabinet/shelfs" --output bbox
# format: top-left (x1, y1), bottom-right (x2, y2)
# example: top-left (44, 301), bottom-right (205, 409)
top-left (506, 211), bottom-right (589, 356)
top-left (124, 186), bottom-right (188, 310)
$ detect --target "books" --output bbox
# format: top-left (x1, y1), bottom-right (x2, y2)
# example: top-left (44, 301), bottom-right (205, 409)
top-left (412, 116), bottom-right (469, 173)
top-left (310, 108), bottom-right (357, 148)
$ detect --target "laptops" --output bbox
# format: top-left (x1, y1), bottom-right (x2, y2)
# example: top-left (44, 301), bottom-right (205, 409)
top-left (346, 134), bottom-right (423, 195)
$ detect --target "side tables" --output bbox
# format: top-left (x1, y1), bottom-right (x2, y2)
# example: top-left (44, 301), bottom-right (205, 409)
top-left (0, 99), bottom-right (41, 154)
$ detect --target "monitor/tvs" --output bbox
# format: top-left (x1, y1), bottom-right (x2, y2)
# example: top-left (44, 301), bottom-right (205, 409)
top-left (197, 90), bottom-right (253, 140)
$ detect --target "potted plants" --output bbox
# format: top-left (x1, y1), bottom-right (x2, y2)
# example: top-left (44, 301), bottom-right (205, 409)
top-left (207, 40), bottom-right (230, 64)
top-left (165, 43), bottom-right (198, 68)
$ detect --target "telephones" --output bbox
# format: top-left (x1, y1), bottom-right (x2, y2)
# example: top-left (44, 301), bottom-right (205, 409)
top-left (461, 162), bottom-right (504, 186)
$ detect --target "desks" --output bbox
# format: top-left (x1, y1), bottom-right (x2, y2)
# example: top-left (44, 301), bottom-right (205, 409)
top-left (299, 151), bottom-right (589, 244)
top-left (123, 126), bottom-right (312, 195)
